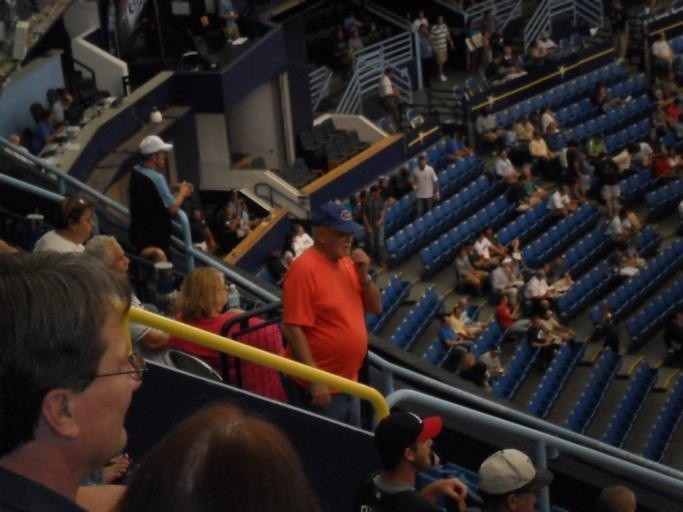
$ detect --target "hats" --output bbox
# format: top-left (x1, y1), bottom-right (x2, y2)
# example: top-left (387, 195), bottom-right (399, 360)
top-left (374, 412), bottom-right (442, 456)
top-left (540, 32), bottom-right (549, 39)
top-left (499, 257), bottom-right (512, 265)
top-left (539, 309), bottom-right (553, 319)
top-left (477, 449), bottom-right (553, 500)
top-left (225, 209), bottom-right (232, 215)
top-left (311, 202), bottom-right (365, 233)
top-left (590, 28), bottom-right (599, 36)
top-left (140, 135), bottom-right (172, 155)
top-left (559, 39), bottom-right (569, 48)
top-left (571, 34), bottom-right (583, 44)
top-left (513, 253), bottom-right (522, 261)
top-left (493, 345), bottom-right (501, 353)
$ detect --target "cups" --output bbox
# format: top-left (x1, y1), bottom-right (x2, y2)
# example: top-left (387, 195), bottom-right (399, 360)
top-left (154, 262), bottom-right (173, 281)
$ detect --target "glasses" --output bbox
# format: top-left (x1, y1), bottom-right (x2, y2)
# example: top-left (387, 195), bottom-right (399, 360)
top-left (112, 253), bottom-right (126, 264)
top-left (75, 197), bottom-right (85, 213)
top-left (515, 489), bottom-right (540, 497)
top-left (58, 352), bottom-right (148, 384)
top-left (219, 284), bottom-right (228, 293)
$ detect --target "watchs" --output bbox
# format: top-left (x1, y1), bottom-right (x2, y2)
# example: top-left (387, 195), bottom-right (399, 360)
top-left (360, 275), bottom-right (375, 289)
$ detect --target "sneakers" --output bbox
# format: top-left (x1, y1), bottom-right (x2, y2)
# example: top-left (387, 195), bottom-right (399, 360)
top-left (546, 40), bottom-right (557, 49)
top-left (614, 57), bottom-right (626, 66)
top-left (438, 74), bottom-right (447, 82)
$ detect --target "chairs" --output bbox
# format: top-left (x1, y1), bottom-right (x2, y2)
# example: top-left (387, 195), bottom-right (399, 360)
top-left (363, 274), bottom-right (683, 461)
top-left (489, 60), bottom-right (682, 221)
top-left (327, 140), bottom-right (682, 347)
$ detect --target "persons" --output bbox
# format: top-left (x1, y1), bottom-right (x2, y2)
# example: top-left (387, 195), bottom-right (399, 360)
top-left (138, 244), bottom-right (169, 264)
top-left (662, 309), bottom-right (683, 361)
top-left (283, 201), bottom-right (385, 433)
top-left (171, 269), bottom-right (287, 402)
top-left (650, 33), bottom-right (683, 139)
top-left (591, 302), bottom-right (614, 341)
top-left (439, 133), bottom-right (471, 171)
top-left (416, 23), bottom-right (433, 87)
top-left (462, 11), bottom-right (586, 85)
top-left (171, 0), bottom-right (242, 71)
top-left (358, 410), bottom-right (467, 512)
top-left (1, 252), bottom-right (139, 512)
top-left (596, 80), bottom-right (622, 112)
top-left (130, 134), bottom-right (193, 261)
top-left (478, 448), bottom-right (555, 511)
top-left (609, 2), bottom-right (628, 64)
top-left (5, 90), bottom-right (76, 162)
top-left (266, 224), bottom-right (314, 276)
top-left (190, 189), bottom-right (262, 254)
top-left (433, 16), bottom-right (455, 82)
top-left (412, 12), bottom-right (428, 31)
top-left (30, 196), bottom-right (94, 254)
top-left (437, 226), bottom-right (577, 393)
top-left (586, 132), bottom-right (683, 285)
top-left (86, 234), bottom-right (172, 371)
top-left (110, 404), bottom-right (322, 512)
top-left (479, 105), bottom-right (590, 217)
top-left (595, 486), bottom-right (638, 511)
top-left (353, 166), bottom-right (409, 271)
top-left (410, 157), bottom-right (441, 220)
top-left (331, 10), bottom-right (382, 79)
top-left (377, 68), bottom-right (401, 124)
